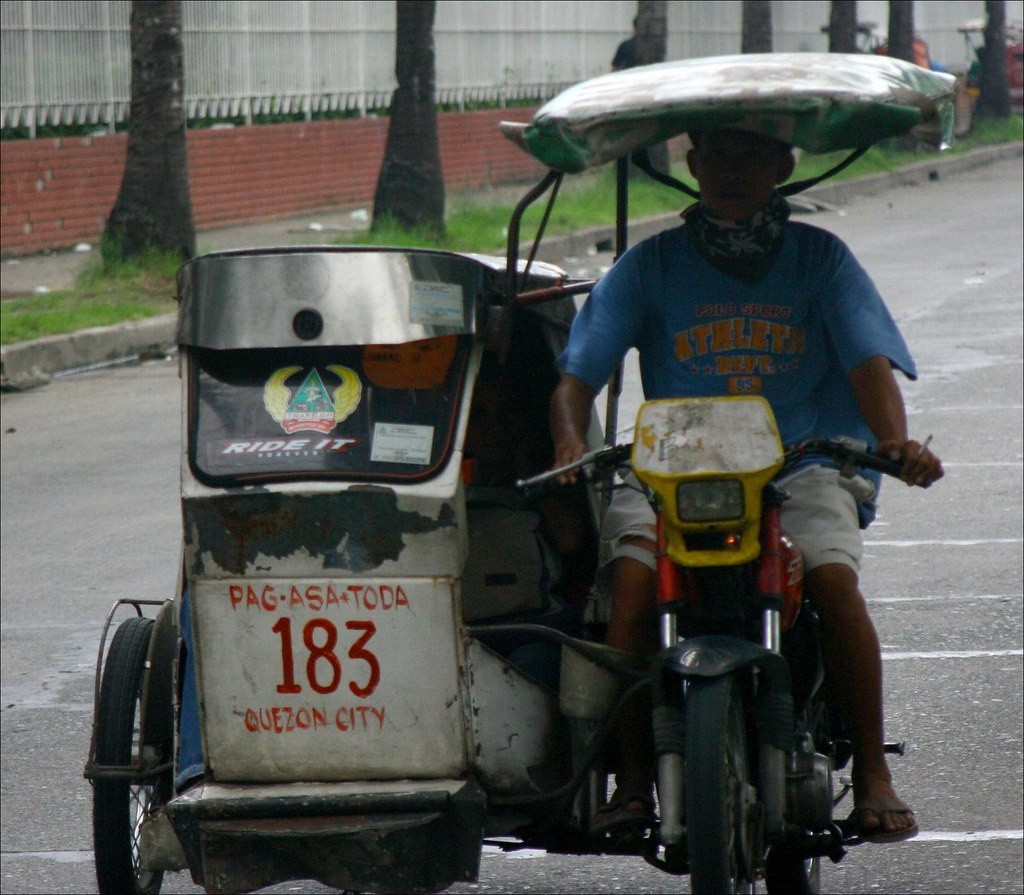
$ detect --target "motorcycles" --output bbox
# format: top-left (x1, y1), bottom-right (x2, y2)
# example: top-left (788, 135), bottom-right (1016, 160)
top-left (817, 11), bottom-right (1024, 135)
top-left (83, 50), bottom-right (949, 895)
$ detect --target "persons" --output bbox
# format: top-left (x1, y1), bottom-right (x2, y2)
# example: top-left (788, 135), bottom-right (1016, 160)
top-left (965, 47), bottom-right (987, 97)
top-left (877, 38), bottom-right (954, 151)
top-left (612, 17), bottom-right (641, 71)
top-left (554, 120), bottom-right (945, 844)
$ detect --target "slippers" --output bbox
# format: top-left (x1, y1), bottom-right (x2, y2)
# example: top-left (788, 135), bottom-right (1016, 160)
top-left (587, 782), bottom-right (659, 838)
top-left (853, 795), bottom-right (919, 844)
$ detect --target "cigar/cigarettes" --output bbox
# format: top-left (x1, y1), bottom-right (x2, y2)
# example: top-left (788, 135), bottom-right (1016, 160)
top-left (919, 432), bottom-right (932, 456)
